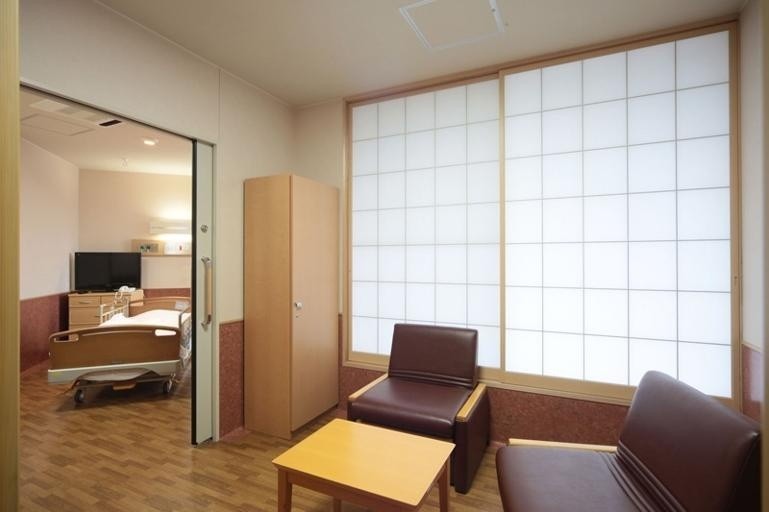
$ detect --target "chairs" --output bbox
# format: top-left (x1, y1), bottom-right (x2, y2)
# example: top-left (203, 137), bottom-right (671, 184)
top-left (347, 322), bottom-right (491, 494)
top-left (494, 368), bottom-right (762, 510)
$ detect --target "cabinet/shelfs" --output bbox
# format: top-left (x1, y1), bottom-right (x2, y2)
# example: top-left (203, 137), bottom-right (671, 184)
top-left (242, 173), bottom-right (340, 440)
top-left (68, 290), bottom-right (143, 341)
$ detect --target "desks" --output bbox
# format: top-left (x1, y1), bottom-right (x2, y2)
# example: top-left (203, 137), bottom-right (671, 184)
top-left (271, 418), bottom-right (456, 511)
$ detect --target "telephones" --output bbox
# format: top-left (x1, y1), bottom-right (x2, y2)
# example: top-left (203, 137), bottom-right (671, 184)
top-left (118, 285), bottom-right (136, 292)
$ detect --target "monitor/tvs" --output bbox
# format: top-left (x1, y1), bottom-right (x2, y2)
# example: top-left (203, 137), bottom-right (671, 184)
top-left (74, 252), bottom-right (141, 291)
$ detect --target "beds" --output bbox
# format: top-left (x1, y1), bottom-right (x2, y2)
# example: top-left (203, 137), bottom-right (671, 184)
top-left (48, 295), bottom-right (191, 402)
